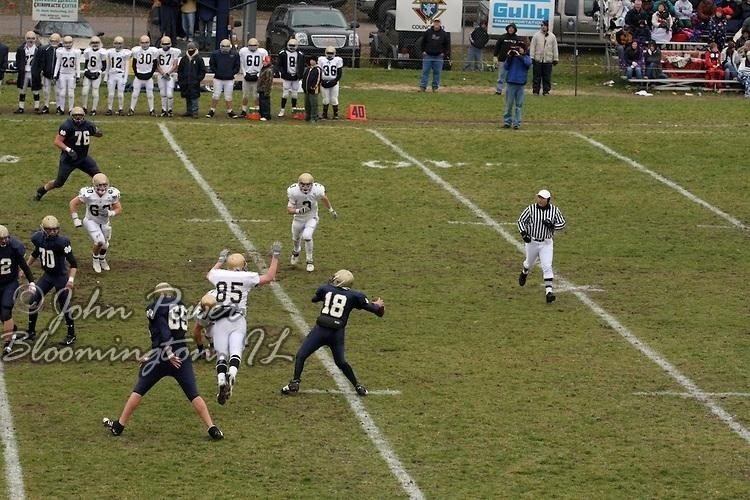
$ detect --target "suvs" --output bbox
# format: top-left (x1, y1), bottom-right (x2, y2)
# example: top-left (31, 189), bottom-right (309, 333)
top-left (267, 4), bottom-right (361, 67)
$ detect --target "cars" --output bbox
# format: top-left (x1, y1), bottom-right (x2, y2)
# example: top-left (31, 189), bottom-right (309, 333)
top-left (368, 9), bottom-right (452, 71)
top-left (32, 18), bottom-right (103, 50)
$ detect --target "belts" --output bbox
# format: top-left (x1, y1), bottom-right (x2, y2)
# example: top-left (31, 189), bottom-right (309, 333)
top-left (534, 239), bottom-right (545, 242)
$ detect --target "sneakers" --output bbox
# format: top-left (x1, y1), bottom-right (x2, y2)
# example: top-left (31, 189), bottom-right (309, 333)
top-left (306, 260), bottom-right (315, 271)
top-left (519, 269), bottom-right (529, 286)
top-left (290, 254), bottom-right (300, 266)
top-left (34, 186), bottom-right (48, 201)
top-left (103, 373), bottom-right (368, 439)
top-left (13, 105), bottom-right (343, 121)
top-left (100, 259), bottom-right (111, 271)
top-left (546, 291), bottom-right (556, 305)
top-left (2, 325), bottom-right (77, 355)
top-left (92, 260), bottom-right (101, 273)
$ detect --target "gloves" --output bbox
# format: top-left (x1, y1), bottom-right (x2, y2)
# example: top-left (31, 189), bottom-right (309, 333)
top-left (542, 219), bottom-right (555, 230)
top-left (73, 218), bottom-right (83, 227)
top-left (327, 206), bottom-right (338, 219)
top-left (520, 230), bottom-right (531, 243)
top-left (295, 205), bottom-right (311, 215)
top-left (217, 247), bottom-right (230, 264)
top-left (270, 240), bottom-right (283, 256)
top-left (65, 147), bottom-right (78, 160)
top-left (97, 207), bottom-right (113, 218)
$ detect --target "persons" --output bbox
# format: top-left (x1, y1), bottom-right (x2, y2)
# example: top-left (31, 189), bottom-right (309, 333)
top-left (35, 106), bottom-right (104, 202)
top-left (0, 1), bottom-right (343, 123)
top-left (465, 18), bottom-right (559, 129)
top-left (517, 190), bottom-right (566, 301)
top-left (103, 243), bottom-right (285, 441)
top-left (69, 173), bottom-right (122, 273)
top-left (287, 173), bottom-right (337, 272)
top-left (592, 1), bottom-right (750, 96)
top-left (0, 225), bottom-right (36, 354)
top-left (282, 269), bottom-right (384, 396)
top-left (419, 19), bottom-right (451, 96)
top-left (17, 216), bottom-right (77, 343)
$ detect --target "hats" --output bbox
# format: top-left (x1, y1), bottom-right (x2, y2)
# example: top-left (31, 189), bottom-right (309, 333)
top-left (536, 190), bottom-right (551, 199)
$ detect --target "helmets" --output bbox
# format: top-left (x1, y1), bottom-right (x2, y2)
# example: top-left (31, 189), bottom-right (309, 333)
top-left (224, 253), bottom-right (249, 272)
top-left (0, 225), bottom-right (11, 248)
top-left (297, 173), bottom-right (314, 194)
top-left (201, 295), bottom-right (218, 313)
top-left (153, 283), bottom-right (176, 301)
top-left (25, 31), bottom-right (337, 60)
top-left (40, 215), bottom-right (61, 240)
top-left (328, 269), bottom-right (354, 288)
top-left (92, 173), bottom-right (110, 195)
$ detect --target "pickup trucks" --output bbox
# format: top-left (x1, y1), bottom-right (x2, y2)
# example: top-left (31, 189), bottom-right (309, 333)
top-left (477, 0), bottom-right (616, 48)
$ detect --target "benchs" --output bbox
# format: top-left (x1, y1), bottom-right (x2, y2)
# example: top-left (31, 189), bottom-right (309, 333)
top-left (629, 40), bottom-right (744, 93)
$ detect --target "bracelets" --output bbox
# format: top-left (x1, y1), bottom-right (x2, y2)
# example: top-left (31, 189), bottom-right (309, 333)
top-left (111, 210), bottom-right (115, 217)
top-left (64, 147), bottom-right (72, 152)
top-left (71, 212), bottom-right (79, 217)
top-left (29, 282), bottom-right (36, 286)
top-left (66, 277), bottom-right (75, 284)
top-left (329, 208), bottom-right (334, 213)
top-left (295, 209), bottom-right (299, 213)
top-left (164, 346), bottom-right (175, 359)
